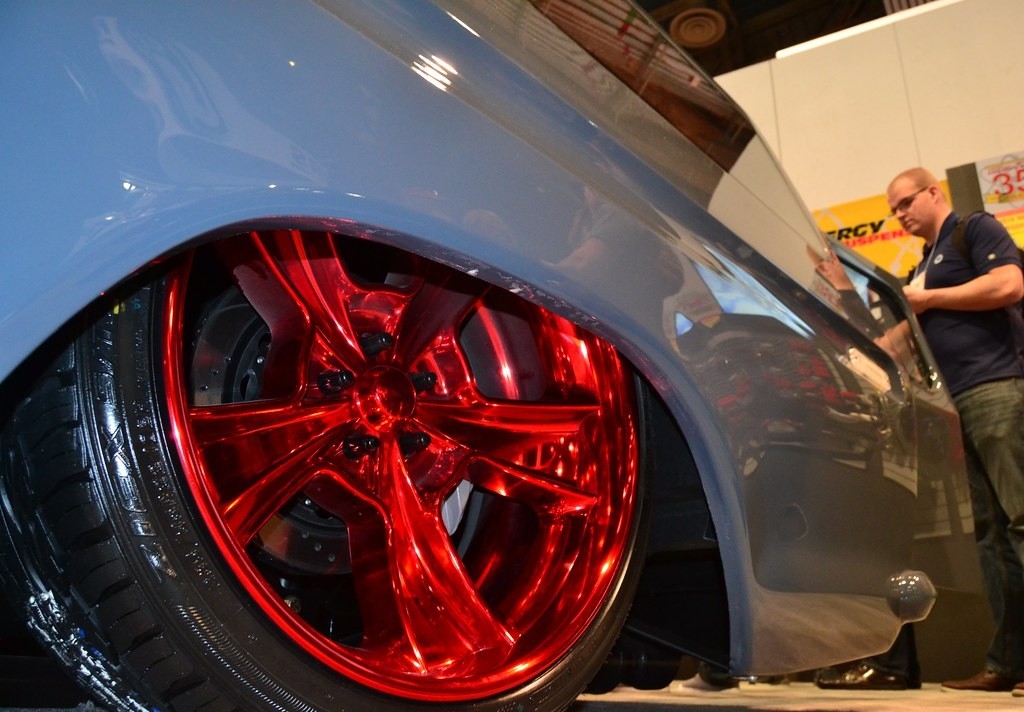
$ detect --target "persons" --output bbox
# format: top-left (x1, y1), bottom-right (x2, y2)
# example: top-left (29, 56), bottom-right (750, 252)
top-left (806, 233), bottom-right (918, 364)
top-left (886, 167), bottom-right (1024, 697)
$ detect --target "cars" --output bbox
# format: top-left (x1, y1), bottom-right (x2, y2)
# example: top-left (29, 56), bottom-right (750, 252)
top-left (0, 0), bottom-right (999, 712)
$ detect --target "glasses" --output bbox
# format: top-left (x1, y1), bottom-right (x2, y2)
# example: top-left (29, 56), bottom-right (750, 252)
top-left (888, 187), bottom-right (928, 217)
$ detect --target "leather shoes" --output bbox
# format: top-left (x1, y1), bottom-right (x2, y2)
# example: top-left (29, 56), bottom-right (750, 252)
top-left (817, 663), bottom-right (909, 689)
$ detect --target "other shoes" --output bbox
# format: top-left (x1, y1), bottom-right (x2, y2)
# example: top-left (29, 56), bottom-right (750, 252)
top-left (1011, 682), bottom-right (1024, 697)
top-left (669, 673), bottom-right (739, 698)
top-left (941, 669), bottom-right (1013, 696)
